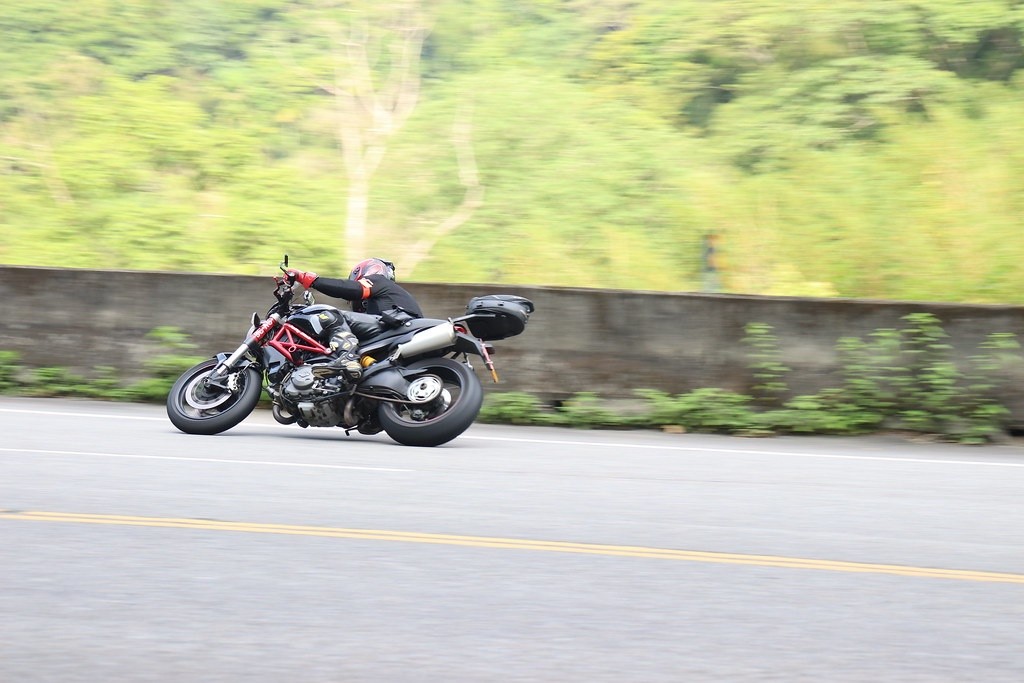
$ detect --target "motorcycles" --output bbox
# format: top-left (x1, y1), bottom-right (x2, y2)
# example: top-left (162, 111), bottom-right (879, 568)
top-left (166, 253), bottom-right (535, 448)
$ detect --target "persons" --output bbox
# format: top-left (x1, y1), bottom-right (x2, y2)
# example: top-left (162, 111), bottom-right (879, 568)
top-left (283, 258), bottom-right (423, 380)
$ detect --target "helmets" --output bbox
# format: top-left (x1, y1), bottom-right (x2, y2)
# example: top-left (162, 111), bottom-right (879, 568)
top-left (348, 257), bottom-right (395, 282)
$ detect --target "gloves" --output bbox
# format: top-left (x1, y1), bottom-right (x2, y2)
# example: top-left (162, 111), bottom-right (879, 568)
top-left (284, 268), bottom-right (318, 289)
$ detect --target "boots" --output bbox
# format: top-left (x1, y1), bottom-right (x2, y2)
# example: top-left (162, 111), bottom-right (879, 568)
top-left (312, 331), bottom-right (362, 379)
